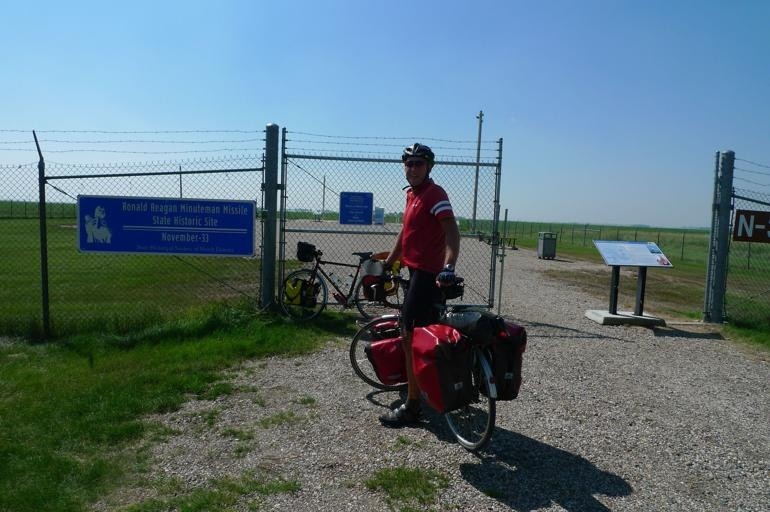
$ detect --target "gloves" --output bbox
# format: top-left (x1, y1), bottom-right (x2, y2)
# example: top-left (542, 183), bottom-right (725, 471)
top-left (436, 263), bottom-right (457, 285)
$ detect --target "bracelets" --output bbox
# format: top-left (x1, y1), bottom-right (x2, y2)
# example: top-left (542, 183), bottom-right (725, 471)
top-left (443, 263), bottom-right (455, 272)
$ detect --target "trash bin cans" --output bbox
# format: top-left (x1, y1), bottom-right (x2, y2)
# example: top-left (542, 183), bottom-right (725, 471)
top-left (537, 232), bottom-right (557, 260)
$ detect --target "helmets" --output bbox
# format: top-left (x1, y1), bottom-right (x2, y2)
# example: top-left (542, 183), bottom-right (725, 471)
top-left (401, 143), bottom-right (435, 168)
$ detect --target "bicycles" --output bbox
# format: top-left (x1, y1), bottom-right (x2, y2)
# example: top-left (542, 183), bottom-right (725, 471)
top-left (280, 242), bottom-right (407, 321)
top-left (349, 258), bottom-right (497, 452)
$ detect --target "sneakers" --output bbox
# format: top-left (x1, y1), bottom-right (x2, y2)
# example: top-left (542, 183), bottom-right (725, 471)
top-left (379, 403), bottom-right (424, 423)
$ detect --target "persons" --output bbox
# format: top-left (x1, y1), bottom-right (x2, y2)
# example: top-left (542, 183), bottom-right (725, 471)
top-left (373, 142), bottom-right (462, 426)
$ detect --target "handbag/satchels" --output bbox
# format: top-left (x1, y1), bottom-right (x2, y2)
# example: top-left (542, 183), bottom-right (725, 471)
top-left (282, 277), bottom-right (322, 309)
top-left (411, 323), bottom-right (476, 415)
top-left (371, 314), bottom-right (403, 336)
top-left (437, 306), bottom-right (504, 344)
top-left (359, 259), bottom-right (388, 302)
top-left (477, 320), bottom-right (527, 400)
top-left (366, 337), bottom-right (409, 385)
